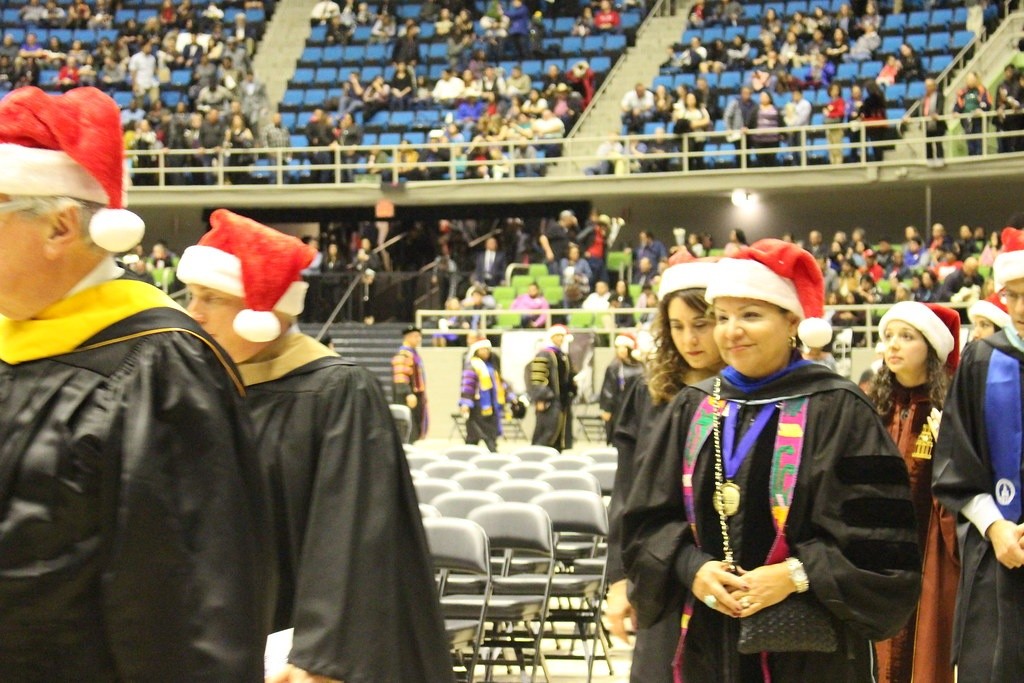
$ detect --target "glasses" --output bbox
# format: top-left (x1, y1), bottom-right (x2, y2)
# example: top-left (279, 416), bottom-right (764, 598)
top-left (998, 287), bottom-right (1024, 306)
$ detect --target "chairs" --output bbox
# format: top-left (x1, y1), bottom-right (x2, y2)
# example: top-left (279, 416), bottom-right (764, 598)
top-left (401, 439), bottom-right (624, 682)
top-left (1, 0), bottom-right (1000, 190)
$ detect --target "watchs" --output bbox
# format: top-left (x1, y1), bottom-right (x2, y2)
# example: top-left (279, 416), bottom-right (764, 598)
top-left (788, 557), bottom-right (810, 593)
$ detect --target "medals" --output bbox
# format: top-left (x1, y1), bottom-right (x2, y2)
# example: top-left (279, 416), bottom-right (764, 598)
top-left (712, 482), bottom-right (741, 514)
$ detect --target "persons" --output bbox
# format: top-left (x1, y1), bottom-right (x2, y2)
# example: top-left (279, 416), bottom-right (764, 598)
top-left (178, 209), bottom-right (453, 683)
top-left (303, 234), bottom-right (379, 325)
top-left (602, 257), bottom-right (728, 682)
top-left (624, 239), bottom-right (922, 682)
top-left (417, 210), bottom-right (1002, 347)
top-left (934, 228), bottom-right (1024, 683)
top-left (0, 86), bottom-right (274, 683)
top-left (113, 238), bottom-right (183, 291)
top-left (531, 323), bottom-right (578, 451)
top-left (599, 335), bottom-right (646, 446)
top-left (394, 325), bottom-right (429, 440)
top-left (1, 1), bottom-right (1024, 182)
top-left (458, 338), bottom-right (517, 451)
top-left (862, 302), bottom-right (963, 683)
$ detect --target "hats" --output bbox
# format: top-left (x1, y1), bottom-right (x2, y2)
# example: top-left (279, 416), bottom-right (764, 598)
top-left (993, 227), bottom-right (1024, 292)
top-left (705, 238), bottom-right (832, 348)
top-left (967, 294), bottom-right (1010, 328)
top-left (656, 248), bottom-right (721, 300)
top-left (614, 334), bottom-right (639, 355)
top-left (549, 323), bottom-right (573, 342)
top-left (876, 301), bottom-right (960, 376)
top-left (402, 326), bottom-right (420, 335)
top-left (0, 86), bottom-right (145, 253)
top-left (470, 338), bottom-right (492, 353)
top-left (178, 208), bottom-right (318, 343)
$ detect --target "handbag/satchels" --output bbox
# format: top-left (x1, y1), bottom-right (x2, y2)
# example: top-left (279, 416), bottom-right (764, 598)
top-left (734, 594), bottom-right (839, 654)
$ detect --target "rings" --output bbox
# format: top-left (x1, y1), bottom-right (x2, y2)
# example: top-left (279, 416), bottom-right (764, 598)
top-left (741, 597), bottom-right (749, 607)
top-left (706, 596), bottom-right (716, 608)
